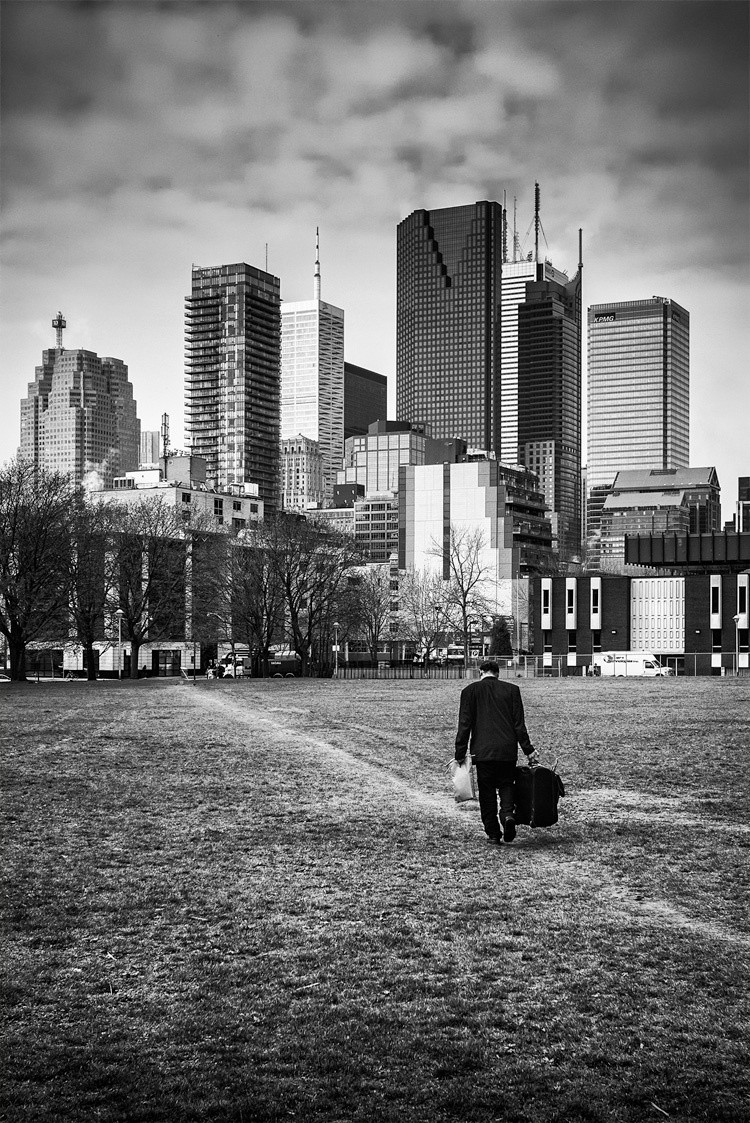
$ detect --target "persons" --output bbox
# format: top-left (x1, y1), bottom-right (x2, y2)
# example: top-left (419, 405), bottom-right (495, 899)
top-left (455, 661), bottom-right (538, 844)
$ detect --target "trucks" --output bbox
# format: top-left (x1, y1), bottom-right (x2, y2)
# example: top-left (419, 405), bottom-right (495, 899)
top-left (212, 648), bottom-right (302, 679)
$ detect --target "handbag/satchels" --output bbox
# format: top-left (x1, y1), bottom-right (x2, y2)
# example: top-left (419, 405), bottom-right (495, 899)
top-left (451, 754), bottom-right (473, 801)
top-left (513, 758), bottom-right (564, 828)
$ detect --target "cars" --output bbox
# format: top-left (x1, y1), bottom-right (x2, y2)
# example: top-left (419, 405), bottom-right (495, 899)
top-left (0, 674), bottom-right (11, 683)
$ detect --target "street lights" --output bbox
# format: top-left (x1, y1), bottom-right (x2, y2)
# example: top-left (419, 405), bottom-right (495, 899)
top-left (116, 609), bottom-right (124, 679)
top-left (733, 614), bottom-right (741, 677)
top-left (333, 620), bottom-right (340, 678)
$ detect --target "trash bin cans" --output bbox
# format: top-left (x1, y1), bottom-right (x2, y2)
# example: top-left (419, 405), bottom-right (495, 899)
top-left (594, 666), bottom-right (601, 676)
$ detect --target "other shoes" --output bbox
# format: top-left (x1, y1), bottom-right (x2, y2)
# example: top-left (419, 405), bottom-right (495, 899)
top-left (487, 836), bottom-right (500, 842)
top-left (504, 816), bottom-right (516, 842)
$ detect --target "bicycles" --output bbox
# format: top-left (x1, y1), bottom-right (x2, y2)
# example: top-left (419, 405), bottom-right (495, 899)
top-left (65, 671), bottom-right (79, 680)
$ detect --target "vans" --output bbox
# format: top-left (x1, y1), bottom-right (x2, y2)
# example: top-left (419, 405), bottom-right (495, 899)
top-left (444, 645), bottom-right (465, 666)
top-left (600, 650), bottom-right (675, 678)
